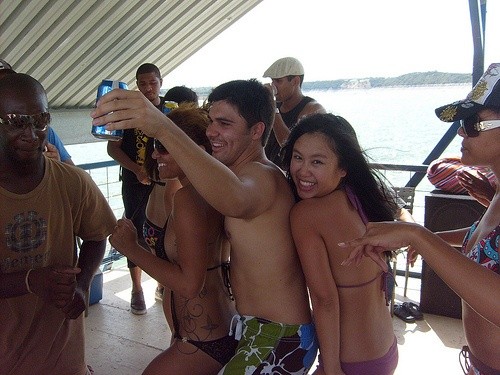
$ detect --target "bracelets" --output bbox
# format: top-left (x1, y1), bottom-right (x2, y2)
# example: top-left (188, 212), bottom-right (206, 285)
top-left (26, 268), bottom-right (34, 294)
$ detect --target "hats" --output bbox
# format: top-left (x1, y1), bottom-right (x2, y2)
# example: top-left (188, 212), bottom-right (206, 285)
top-left (262, 57), bottom-right (304, 78)
top-left (435, 63), bottom-right (500, 122)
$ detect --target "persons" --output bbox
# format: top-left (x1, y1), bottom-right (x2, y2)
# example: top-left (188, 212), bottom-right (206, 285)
top-left (0, 74), bottom-right (119, 375)
top-left (0, 68), bottom-right (75, 167)
top-left (338, 64), bottom-right (500, 375)
top-left (92, 78), bottom-right (318, 375)
top-left (455, 168), bottom-right (496, 209)
top-left (109, 108), bottom-right (242, 375)
top-left (276, 112), bottom-right (402, 375)
top-left (264, 57), bottom-right (327, 170)
top-left (107, 63), bottom-right (198, 315)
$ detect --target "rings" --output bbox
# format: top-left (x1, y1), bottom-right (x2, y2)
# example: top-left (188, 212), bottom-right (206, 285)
top-left (114, 225), bottom-right (118, 231)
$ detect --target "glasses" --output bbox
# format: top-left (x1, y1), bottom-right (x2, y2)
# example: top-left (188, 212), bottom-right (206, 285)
top-left (0, 112), bottom-right (52, 132)
top-left (155, 139), bottom-right (170, 154)
top-left (459, 113), bottom-right (500, 137)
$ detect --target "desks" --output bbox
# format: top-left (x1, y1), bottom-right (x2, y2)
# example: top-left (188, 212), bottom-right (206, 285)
top-left (419, 190), bottom-right (484, 319)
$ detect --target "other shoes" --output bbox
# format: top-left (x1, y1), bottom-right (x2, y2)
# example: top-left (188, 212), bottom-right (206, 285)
top-left (130, 287), bottom-right (146, 315)
top-left (155, 286), bottom-right (164, 301)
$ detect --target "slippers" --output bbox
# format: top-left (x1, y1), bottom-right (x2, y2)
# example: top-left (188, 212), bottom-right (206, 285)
top-left (402, 301), bottom-right (424, 320)
top-left (393, 304), bottom-right (415, 323)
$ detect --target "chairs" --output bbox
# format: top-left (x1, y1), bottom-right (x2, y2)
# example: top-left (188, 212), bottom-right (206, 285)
top-left (392, 187), bottom-right (415, 305)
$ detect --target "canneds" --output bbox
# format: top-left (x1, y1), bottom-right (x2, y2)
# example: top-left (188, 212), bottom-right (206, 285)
top-left (91, 80), bottom-right (129, 141)
top-left (162, 101), bottom-right (178, 115)
